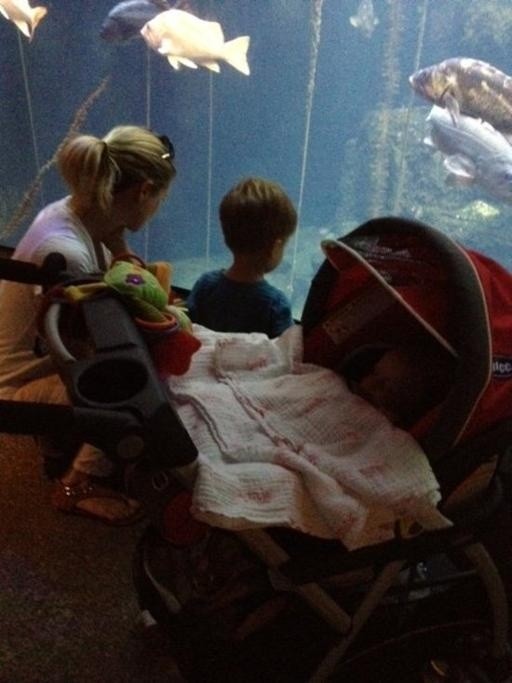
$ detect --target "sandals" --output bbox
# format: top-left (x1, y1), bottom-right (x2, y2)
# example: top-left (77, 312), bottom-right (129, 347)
top-left (52, 475), bottom-right (145, 525)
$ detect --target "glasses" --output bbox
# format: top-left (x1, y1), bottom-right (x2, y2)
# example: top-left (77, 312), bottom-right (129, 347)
top-left (160, 134), bottom-right (176, 161)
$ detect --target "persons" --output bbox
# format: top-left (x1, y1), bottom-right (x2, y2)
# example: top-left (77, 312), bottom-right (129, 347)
top-left (359, 346), bottom-right (423, 412)
top-left (186, 177), bottom-right (297, 339)
top-left (0, 126), bottom-right (176, 528)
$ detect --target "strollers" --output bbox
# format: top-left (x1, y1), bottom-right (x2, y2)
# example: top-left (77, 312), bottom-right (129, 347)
top-left (1, 221), bottom-right (511, 683)
